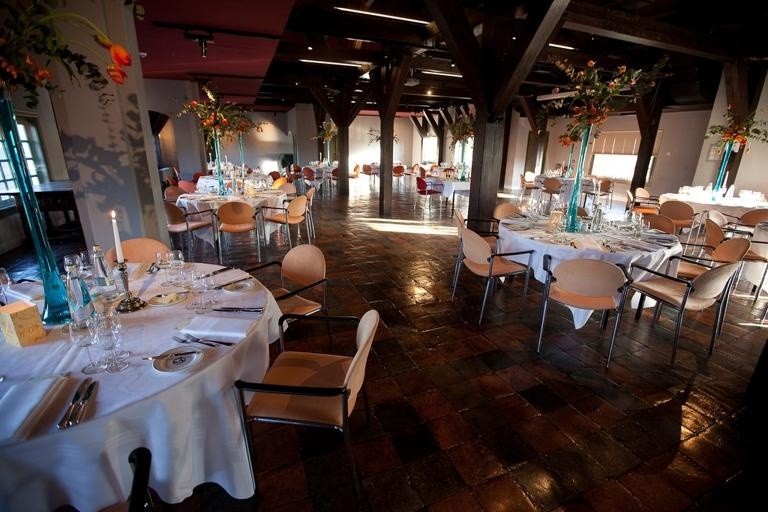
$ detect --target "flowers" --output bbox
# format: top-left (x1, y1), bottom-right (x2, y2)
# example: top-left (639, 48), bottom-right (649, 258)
top-left (1, 0), bottom-right (137, 156)
top-left (229, 107), bottom-right (268, 156)
top-left (172, 83), bottom-right (240, 157)
top-left (312, 118), bottom-right (338, 141)
top-left (700, 101), bottom-right (767, 156)
top-left (445, 114), bottom-right (474, 156)
top-left (530, 51), bottom-right (666, 156)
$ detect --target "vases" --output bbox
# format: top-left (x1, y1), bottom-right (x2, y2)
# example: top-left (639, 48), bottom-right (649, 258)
top-left (565, 143), bottom-right (575, 176)
top-left (0, 99), bottom-right (79, 330)
top-left (459, 140), bottom-right (465, 164)
top-left (238, 133), bottom-right (243, 167)
top-left (211, 129), bottom-right (228, 196)
top-left (712, 140), bottom-right (734, 194)
top-left (562, 123), bottom-right (590, 233)
top-left (321, 141), bottom-right (330, 157)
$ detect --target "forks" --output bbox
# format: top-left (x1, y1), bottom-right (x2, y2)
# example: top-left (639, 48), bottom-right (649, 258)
top-left (169, 332), bottom-right (238, 350)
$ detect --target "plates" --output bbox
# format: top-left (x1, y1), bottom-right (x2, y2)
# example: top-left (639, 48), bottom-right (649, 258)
top-left (225, 280), bottom-right (255, 294)
top-left (146, 292), bottom-right (186, 307)
top-left (152, 346), bottom-right (206, 374)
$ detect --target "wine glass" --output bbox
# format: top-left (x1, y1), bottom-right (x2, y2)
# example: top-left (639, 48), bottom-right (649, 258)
top-left (64, 250), bottom-right (97, 291)
top-left (68, 305), bottom-right (130, 376)
top-left (547, 204), bottom-right (570, 246)
top-left (624, 208), bottom-right (649, 241)
top-left (0, 266), bottom-right (9, 307)
top-left (155, 248), bottom-right (187, 288)
top-left (181, 268), bottom-right (220, 315)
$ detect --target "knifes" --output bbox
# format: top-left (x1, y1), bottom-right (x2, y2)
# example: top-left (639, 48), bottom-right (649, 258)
top-left (141, 351), bottom-right (198, 361)
top-left (55, 375), bottom-right (100, 432)
top-left (212, 304), bottom-right (264, 315)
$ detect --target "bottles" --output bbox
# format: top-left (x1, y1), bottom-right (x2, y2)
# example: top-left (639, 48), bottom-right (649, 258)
top-left (92, 244), bottom-right (118, 298)
top-left (61, 263), bottom-right (99, 330)
top-left (589, 202), bottom-right (604, 233)
